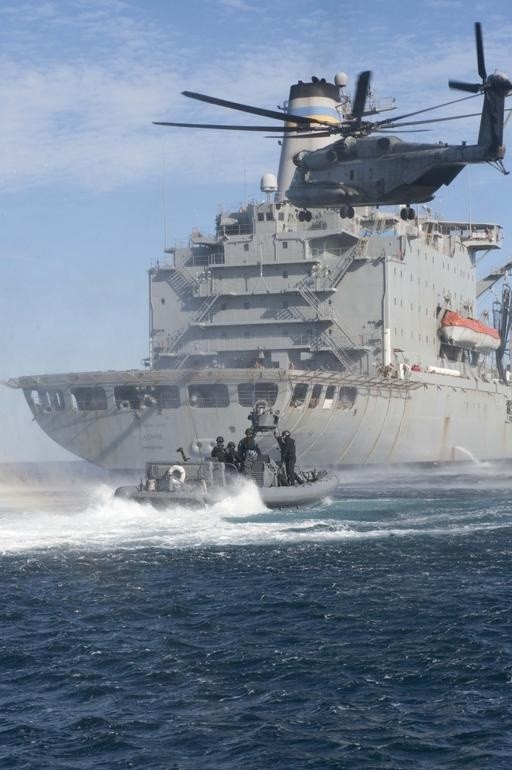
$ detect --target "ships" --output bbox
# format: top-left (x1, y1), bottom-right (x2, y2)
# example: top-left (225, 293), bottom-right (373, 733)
top-left (0, 71), bottom-right (512, 483)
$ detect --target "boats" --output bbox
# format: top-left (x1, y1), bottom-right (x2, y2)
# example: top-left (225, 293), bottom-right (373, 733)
top-left (113, 464), bottom-right (341, 509)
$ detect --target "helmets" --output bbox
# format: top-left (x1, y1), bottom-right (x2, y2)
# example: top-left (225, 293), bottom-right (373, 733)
top-left (227, 442), bottom-right (235, 448)
top-left (216, 437), bottom-right (223, 442)
top-left (246, 429), bottom-right (254, 434)
top-left (282, 431), bottom-right (290, 436)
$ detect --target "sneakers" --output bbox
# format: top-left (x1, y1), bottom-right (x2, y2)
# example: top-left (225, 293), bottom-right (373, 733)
top-left (299, 479), bottom-right (304, 484)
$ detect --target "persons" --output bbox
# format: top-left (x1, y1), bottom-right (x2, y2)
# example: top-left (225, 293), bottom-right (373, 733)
top-left (273, 431), bottom-right (304, 486)
top-left (227, 441), bottom-right (244, 472)
top-left (211, 436), bottom-right (233, 463)
top-left (238, 428), bottom-right (261, 460)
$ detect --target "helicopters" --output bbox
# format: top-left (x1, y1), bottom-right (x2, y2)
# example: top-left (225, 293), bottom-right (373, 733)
top-left (151, 22), bottom-right (511, 225)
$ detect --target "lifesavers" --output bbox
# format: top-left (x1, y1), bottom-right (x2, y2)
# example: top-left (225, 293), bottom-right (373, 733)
top-left (168, 465), bottom-right (186, 486)
